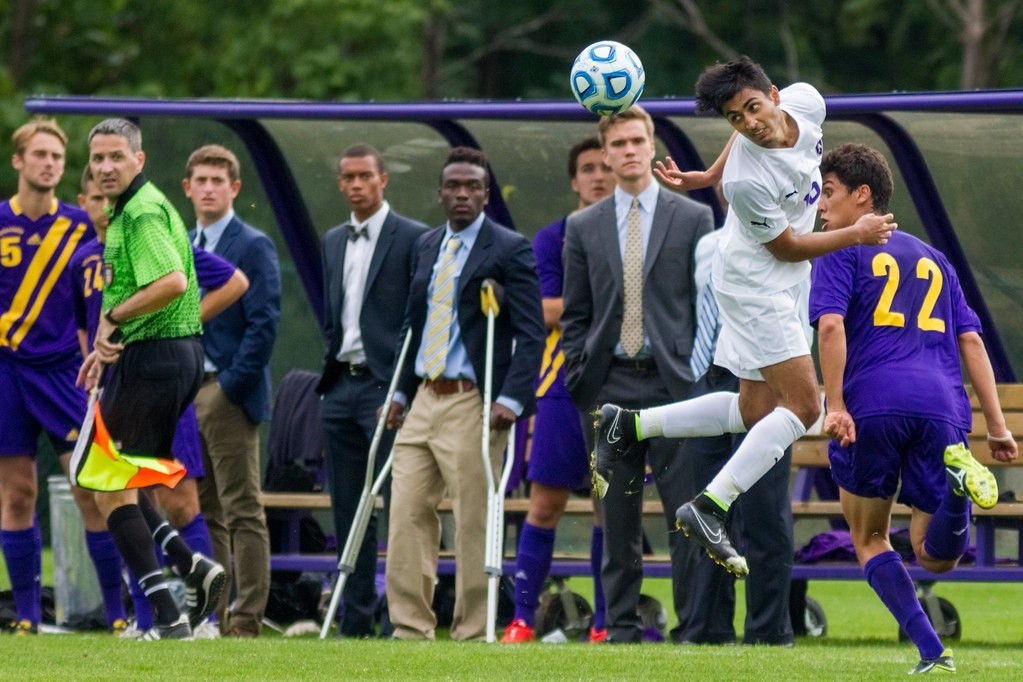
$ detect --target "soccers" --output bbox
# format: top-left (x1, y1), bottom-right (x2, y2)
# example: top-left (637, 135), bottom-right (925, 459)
top-left (570, 40), bottom-right (647, 116)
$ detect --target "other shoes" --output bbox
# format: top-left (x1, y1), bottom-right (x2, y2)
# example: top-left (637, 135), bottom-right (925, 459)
top-left (120, 622), bottom-right (143, 638)
top-left (182, 614), bottom-right (223, 640)
top-left (225, 627), bottom-right (256, 638)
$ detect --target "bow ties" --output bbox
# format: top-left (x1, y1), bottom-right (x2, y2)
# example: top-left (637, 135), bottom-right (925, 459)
top-left (345, 224), bottom-right (369, 241)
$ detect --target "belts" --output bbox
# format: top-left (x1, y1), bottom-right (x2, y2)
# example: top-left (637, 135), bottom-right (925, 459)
top-left (337, 362), bottom-right (374, 377)
top-left (424, 378), bottom-right (478, 395)
top-left (202, 371), bottom-right (218, 383)
top-left (610, 357), bottom-right (658, 371)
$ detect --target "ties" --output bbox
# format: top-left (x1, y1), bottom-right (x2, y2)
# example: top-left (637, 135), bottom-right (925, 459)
top-left (423, 237), bottom-right (462, 382)
top-left (690, 272), bottom-right (719, 382)
top-left (619, 197), bottom-right (645, 359)
top-left (197, 231), bottom-right (206, 250)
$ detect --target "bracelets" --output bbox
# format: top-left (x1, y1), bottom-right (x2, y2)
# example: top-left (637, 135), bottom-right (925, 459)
top-left (987, 430), bottom-right (1012, 442)
top-left (103, 308), bottom-right (120, 326)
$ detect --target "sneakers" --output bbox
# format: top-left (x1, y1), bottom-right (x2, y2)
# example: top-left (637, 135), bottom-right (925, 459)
top-left (907, 649), bottom-right (956, 675)
top-left (590, 402), bottom-right (632, 500)
top-left (943, 441), bottom-right (998, 510)
top-left (108, 618), bottom-right (129, 639)
top-left (589, 626), bottom-right (607, 642)
top-left (135, 614), bottom-right (194, 642)
top-left (499, 619), bottom-right (535, 643)
top-left (674, 500), bottom-right (749, 578)
top-left (8, 620), bottom-right (38, 637)
top-left (172, 552), bottom-right (226, 627)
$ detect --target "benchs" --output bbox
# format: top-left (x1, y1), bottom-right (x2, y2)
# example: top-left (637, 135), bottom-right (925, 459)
top-left (260, 383), bottom-right (1023, 583)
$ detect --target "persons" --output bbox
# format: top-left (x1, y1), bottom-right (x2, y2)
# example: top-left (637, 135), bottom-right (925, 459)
top-left (558, 54), bottom-right (1018, 674)
top-left (181, 144), bottom-right (283, 638)
top-left (77, 154), bottom-right (250, 643)
top-left (501, 135), bottom-right (618, 645)
top-left (376, 145), bottom-right (547, 642)
top-left (0, 119), bottom-right (226, 642)
top-left (312, 143), bottom-right (432, 636)
